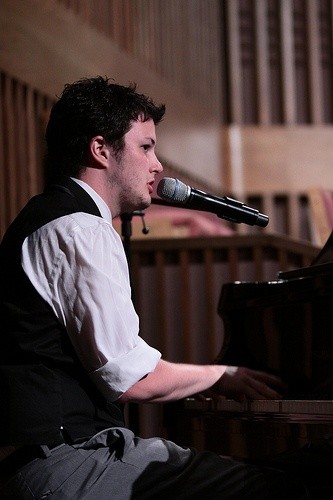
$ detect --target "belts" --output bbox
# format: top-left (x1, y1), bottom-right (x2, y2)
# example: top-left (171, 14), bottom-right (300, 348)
top-left (0, 426), bottom-right (70, 486)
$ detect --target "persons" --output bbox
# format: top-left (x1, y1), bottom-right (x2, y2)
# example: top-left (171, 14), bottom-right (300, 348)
top-left (0, 75), bottom-right (298, 500)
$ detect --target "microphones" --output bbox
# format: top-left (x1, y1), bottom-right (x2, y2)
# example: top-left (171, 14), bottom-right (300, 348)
top-left (156, 177), bottom-right (269, 228)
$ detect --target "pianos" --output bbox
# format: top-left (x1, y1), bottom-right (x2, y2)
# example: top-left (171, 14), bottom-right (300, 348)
top-left (162, 228), bottom-right (333, 499)
